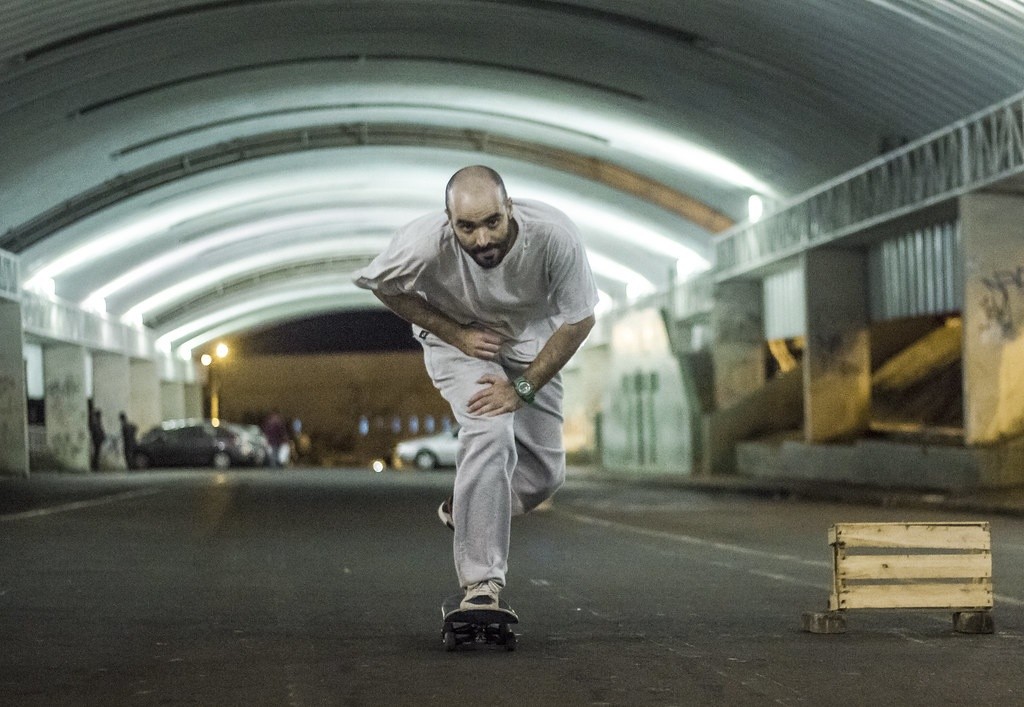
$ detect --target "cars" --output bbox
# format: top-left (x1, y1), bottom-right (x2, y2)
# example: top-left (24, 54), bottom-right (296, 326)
top-left (133, 419), bottom-right (257, 469)
top-left (396, 423), bottom-right (462, 472)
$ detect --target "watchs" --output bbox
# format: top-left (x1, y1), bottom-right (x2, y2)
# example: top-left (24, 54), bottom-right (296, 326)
top-left (512, 375), bottom-right (535, 404)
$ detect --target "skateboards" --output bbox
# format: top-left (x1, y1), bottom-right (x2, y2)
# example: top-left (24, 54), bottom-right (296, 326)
top-left (438, 590), bottom-right (523, 653)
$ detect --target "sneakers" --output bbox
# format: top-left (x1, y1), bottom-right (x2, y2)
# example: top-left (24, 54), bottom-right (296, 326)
top-left (460, 579), bottom-right (503, 610)
top-left (438, 497), bottom-right (454, 532)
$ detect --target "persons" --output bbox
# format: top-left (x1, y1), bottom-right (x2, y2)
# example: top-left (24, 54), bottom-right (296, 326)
top-left (92, 406), bottom-right (106, 472)
top-left (350, 165), bottom-right (597, 611)
top-left (263, 409), bottom-right (292, 468)
top-left (117, 412), bottom-right (140, 470)
